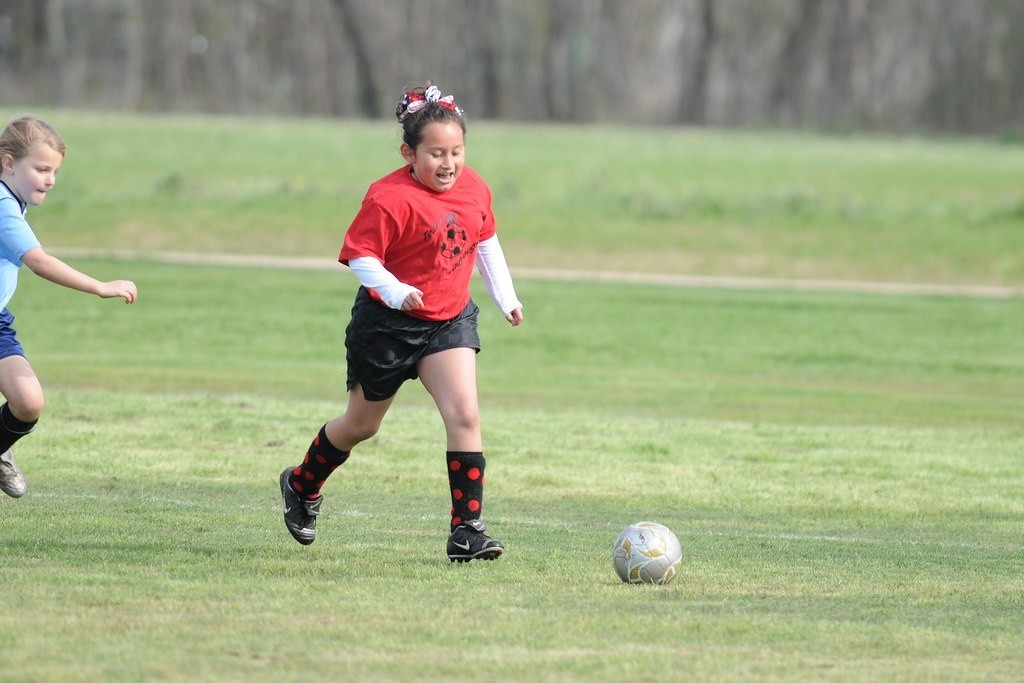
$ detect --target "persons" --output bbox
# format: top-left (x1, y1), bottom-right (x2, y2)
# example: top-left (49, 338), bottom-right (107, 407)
top-left (279, 87), bottom-right (523, 561)
top-left (0, 117), bottom-right (136, 497)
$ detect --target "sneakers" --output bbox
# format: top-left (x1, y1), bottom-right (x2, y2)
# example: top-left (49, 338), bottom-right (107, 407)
top-left (0, 449), bottom-right (28, 499)
top-left (279, 466), bottom-right (324, 545)
top-left (447, 516), bottom-right (504, 563)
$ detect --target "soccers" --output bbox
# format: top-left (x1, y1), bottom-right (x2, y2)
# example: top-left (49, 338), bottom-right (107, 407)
top-left (610, 521), bottom-right (684, 587)
